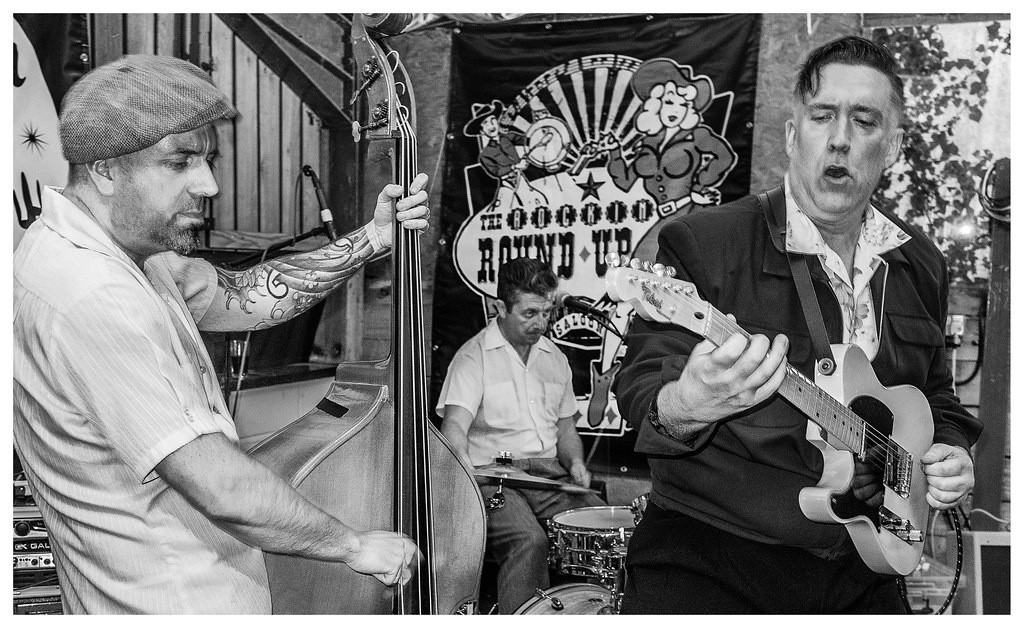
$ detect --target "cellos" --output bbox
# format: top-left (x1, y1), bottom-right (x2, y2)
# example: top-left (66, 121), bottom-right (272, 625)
top-left (231, 24), bottom-right (491, 615)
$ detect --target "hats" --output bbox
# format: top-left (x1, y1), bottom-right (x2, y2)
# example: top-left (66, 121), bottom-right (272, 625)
top-left (59, 54), bottom-right (237, 162)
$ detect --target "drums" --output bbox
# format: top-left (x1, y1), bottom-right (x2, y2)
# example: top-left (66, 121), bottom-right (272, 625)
top-left (545, 505), bottom-right (644, 578)
top-left (511, 583), bottom-right (622, 616)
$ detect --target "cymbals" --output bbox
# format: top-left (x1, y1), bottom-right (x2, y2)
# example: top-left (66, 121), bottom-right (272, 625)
top-left (468, 463), bottom-right (565, 493)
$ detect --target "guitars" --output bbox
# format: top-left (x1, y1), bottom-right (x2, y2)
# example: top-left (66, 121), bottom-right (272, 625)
top-left (601, 251), bottom-right (938, 577)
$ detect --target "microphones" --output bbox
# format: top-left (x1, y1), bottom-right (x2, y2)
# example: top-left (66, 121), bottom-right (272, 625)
top-left (555, 290), bottom-right (605, 317)
top-left (310, 170), bottom-right (338, 242)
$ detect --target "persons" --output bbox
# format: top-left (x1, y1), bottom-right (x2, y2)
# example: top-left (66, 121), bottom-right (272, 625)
top-left (435, 257), bottom-right (609, 615)
top-left (611, 35), bottom-right (983, 616)
top-left (13, 53), bottom-right (431, 615)
